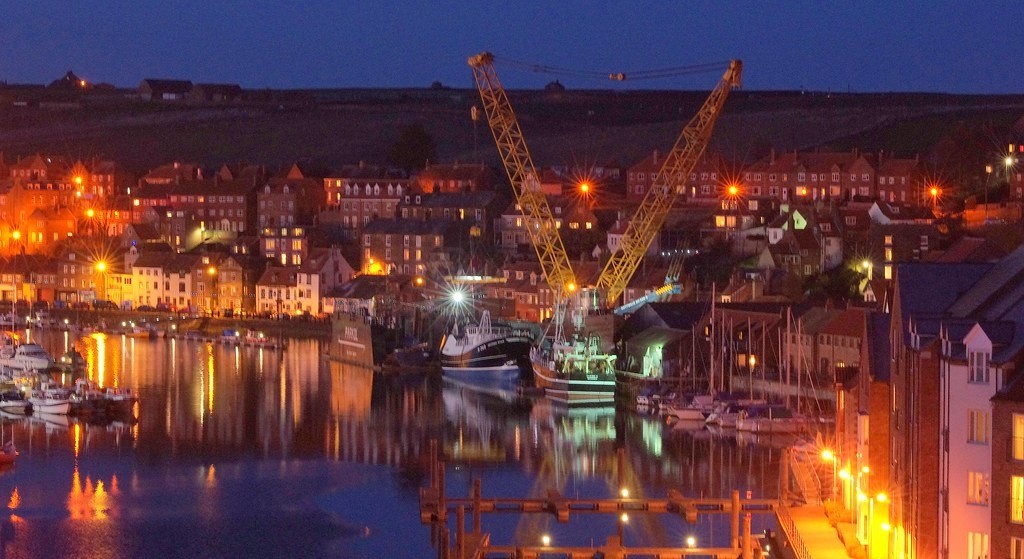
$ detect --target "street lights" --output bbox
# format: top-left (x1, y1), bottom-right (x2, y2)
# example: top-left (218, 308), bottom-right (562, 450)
top-left (820, 448), bottom-right (836, 505)
top-left (839, 471), bottom-right (854, 527)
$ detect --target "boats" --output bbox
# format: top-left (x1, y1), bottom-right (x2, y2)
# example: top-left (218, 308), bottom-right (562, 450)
top-left (618, 279), bottom-right (835, 433)
top-left (0, 270), bottom-right (137, 425)
top-left (530, 285), bottom-right (616, 406)
top-left (442, 311), bottom-right (542, 404)
top-left (125, 323), bottom-right (164, 338)
top-left (0, 441), bottom-right (17, 465)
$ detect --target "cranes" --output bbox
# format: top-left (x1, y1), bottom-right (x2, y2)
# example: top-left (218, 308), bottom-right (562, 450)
top-left (468, 52), bottom-right (744, 312)
